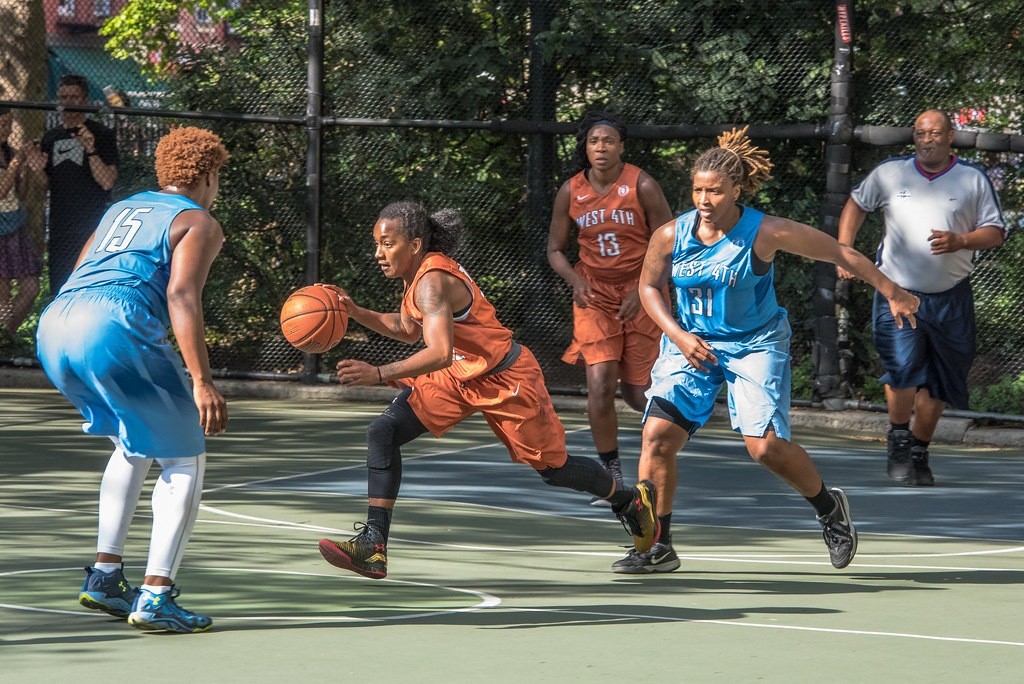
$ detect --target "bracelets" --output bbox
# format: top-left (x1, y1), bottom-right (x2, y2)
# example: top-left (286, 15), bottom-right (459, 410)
top-left (86, 149), bottom-right (99, 158)
top-left (961, 234), bottom-right (968, 246)
top-left (376, 366), bottom-right (382, 382)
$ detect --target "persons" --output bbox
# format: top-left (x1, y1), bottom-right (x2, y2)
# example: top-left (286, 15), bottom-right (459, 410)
top-left (611, 123), bottom-right (920, 572)
top-left (25, 74), bottom-right (121, 293)
top-left (35, 121), bottom-right (229, 630)
top-left (0, 103), bottom-right (41, 364)
top-left (548, 112), bottom-right (671, 484)
top-left (311, 201), bottom-right (661, 580)
top-left (835, 108), bottom-right (1006, 485)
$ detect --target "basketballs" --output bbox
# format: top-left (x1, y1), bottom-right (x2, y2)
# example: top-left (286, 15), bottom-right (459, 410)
top-left (279, 285), bottom-right (348, 355)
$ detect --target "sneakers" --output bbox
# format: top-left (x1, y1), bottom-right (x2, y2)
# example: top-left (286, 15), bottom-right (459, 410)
top-left (814, 487), bottom-right (857, 569)
top-left (907, 440), bottom-right (934, 488)
top-left (610, 530), bottom-right (683, 572)
top-left (589, 456), bottom-right (623, 505)
top-left (128, 587), bottom-right (212, 634)
top-left (886, 423), bottom-right (913, 482)
top-left (611, 475), bottom-right (660, 552)
top-left (80, 564), bottom-right (141, 617)
top-left (319, 521), bottom-right (386, 581)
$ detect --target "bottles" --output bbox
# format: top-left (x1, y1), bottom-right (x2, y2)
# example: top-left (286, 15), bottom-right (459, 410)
top-left (104, 84), bottom-right (127, 121)
